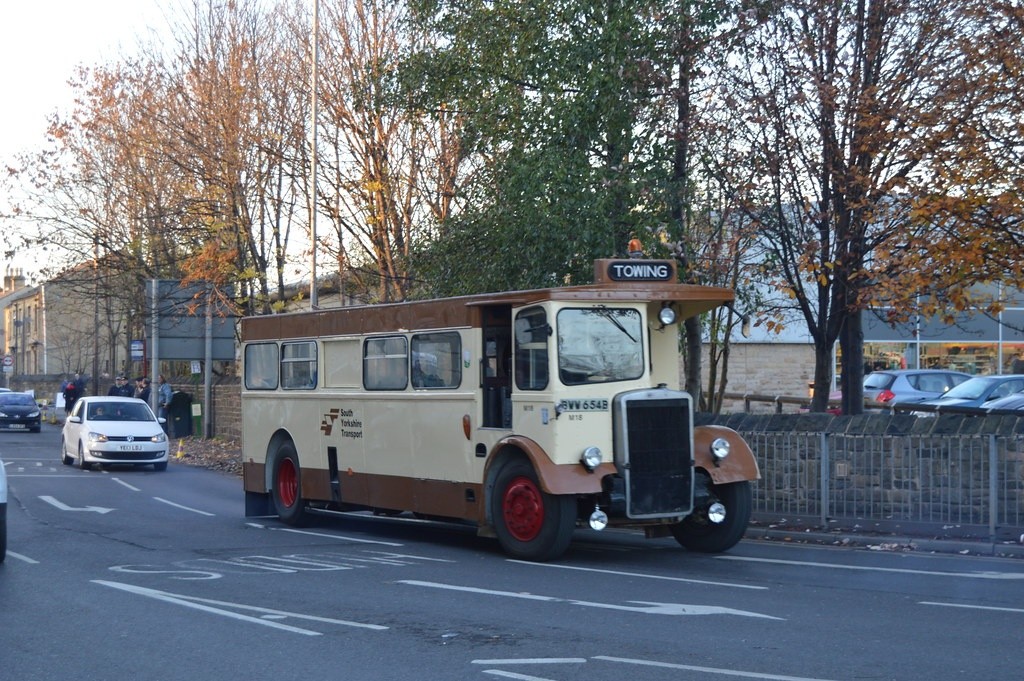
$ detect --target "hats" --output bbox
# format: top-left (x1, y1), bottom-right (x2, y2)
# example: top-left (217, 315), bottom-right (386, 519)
top-left (116, 377), bottom-right (128, 380)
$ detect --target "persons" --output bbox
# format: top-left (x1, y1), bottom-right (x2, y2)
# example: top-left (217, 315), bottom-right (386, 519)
top-left (61, 374), bottom-right (85, 418)
top-left (90, 405), bottom-right (105, 420)
top-left (108, 374), bottom-right (173, 433)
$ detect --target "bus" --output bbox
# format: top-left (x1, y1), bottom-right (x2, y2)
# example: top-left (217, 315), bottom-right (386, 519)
top-left (240, 237), bottom-right (762, 562)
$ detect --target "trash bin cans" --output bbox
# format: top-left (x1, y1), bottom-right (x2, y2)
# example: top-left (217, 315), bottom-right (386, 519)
top-left (808, 381), bottom-right (815, 399)
top-left (165, 391), bottom-right (192, 438)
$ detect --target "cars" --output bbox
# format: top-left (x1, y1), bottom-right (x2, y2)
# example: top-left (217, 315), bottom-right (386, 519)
top-left (863, 369), bottom-right (973, 413)
top-left (0, 388), bottom-right (12, 392)
top-left (0, 392), bottom-right (43, 433)
top-left (910, 374), bottom-right (1024, 418)
top-left (979, 389), bottom-right (1024, 411)
top-left (62, 396), bottom-right (169, 472)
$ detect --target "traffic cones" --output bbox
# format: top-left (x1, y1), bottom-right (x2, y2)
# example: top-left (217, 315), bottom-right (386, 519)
top-left (51, 411), bottom-right (57, 424)
top-left (176, 438), bottom-right (185, 458)
top-left (42, 411), bottom-right (47, 422)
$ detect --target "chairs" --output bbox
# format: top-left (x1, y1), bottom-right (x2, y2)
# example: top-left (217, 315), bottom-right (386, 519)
top-left (933, 381), bottom-right (942, 392)
top-left (918, 380), bottom-right (926, 390)
